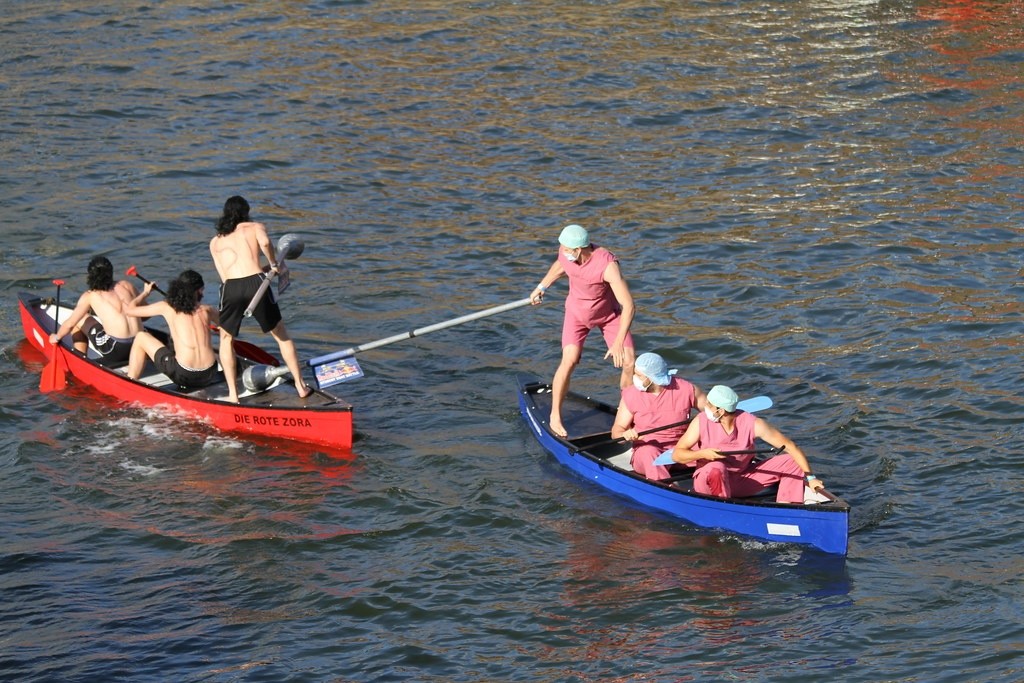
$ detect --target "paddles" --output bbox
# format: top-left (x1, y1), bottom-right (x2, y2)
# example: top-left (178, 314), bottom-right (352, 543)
top-left (653, 444), bottom-right (786, 466)
top-left (125, 266), bottom-right (281, 367)
top-left (39, 280), bottom-right (66, 392)
top-left (568, 395), bottom-right (772, 456)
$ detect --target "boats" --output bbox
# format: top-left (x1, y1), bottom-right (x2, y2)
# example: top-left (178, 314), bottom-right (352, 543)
top-left (516, 368), bottom-right (852, 555)
top-left (15, 289), bottom-right (352, 453)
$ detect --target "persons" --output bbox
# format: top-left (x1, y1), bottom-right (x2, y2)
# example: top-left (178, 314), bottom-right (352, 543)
top-left (529, 224), bottom-right (635, 436)
top-left (125, 270), bottom-right (219, 389)
top-left (49, 257), bottom-right (150, 362)
top-left (610, 352), bottom-right (708, 486)
top-left (209, 196), bottom-right (312, 404)
top-left (670, 384), bottom-right (824, 504)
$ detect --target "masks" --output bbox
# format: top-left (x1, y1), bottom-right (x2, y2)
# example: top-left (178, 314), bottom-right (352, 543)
top-left (564, 252), bottom-right (580, 261)
top-left (632, 375), bottom-right (648, 392)
top-left (704, 407), bottom-right (720, 423)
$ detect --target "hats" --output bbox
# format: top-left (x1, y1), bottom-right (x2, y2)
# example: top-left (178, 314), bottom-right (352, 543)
top-left (558, 225), bottom-right (590, 249)
top-left (635, 353), bottom-right (672, 386)
top-left (707, 385), bottom-right (739, 412)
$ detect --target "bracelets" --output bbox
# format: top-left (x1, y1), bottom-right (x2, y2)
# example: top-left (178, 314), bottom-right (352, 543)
top-left (537, 283), bottom-right (548, 292)
top-left (804, 474), bottom-right (816, 481)
top-left (54, 335), bottom-right (59, 342)
